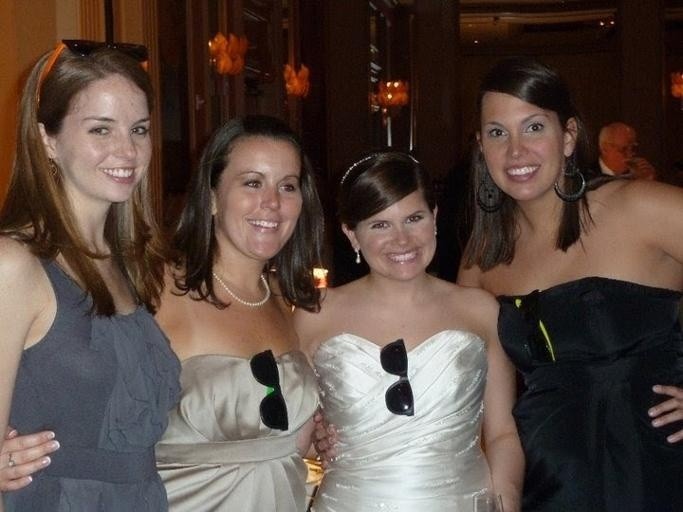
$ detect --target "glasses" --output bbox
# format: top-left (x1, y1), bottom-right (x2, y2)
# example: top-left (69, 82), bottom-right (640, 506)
top-left (380, 338), bottom-right (415, 416)
top-left (61, 41), bottom-right (150, 61)
top-left (250, 348), bottom-right (290, 431)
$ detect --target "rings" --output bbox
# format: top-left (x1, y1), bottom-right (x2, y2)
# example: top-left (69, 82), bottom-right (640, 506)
top-left (7, 452), bottom-right (15, 468)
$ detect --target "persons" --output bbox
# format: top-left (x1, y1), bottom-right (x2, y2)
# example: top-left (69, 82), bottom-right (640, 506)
top-left (295, 143), bottom-right (529, 510)
top-left (144, 110), bottom-right (318, 512)
top-left (0, 40), bottom-right (183, 511)
top-left (309, 57), bottom-right (682, 511)
top-left (437, 115), bottom-right (483, 286)
top-left (584, 121), bottom-right (656, 181)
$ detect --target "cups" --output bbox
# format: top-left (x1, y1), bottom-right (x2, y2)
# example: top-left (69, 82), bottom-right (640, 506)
top-left (474, 491), bottom-right (505, 512)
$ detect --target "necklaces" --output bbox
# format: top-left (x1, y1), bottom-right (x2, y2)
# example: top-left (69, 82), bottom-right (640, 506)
top-left (210, 263), bottom-right (273, 306)
